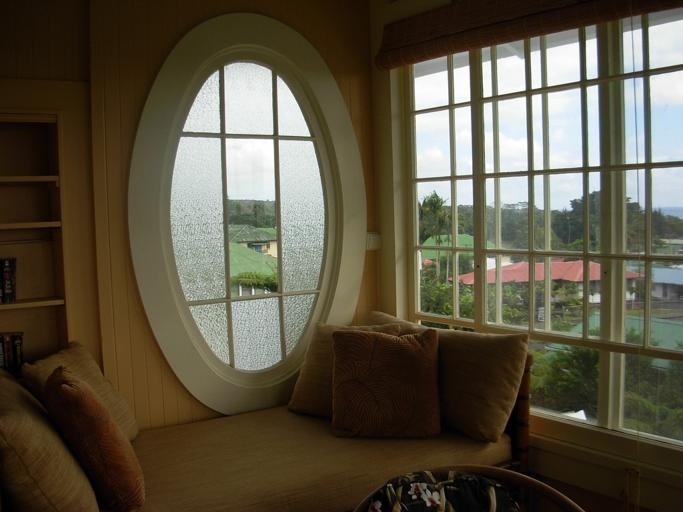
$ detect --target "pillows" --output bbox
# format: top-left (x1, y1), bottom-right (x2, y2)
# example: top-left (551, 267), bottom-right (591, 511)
top-left (369, 310), bottom-right (427, 336)
top-left (45, 365), bottom-right (145, 512)
top-left (0, 376), bottom-right (99, 512)
top-left (332, 330), bottom-right (440, 438)
top-left (428, 326), bottom-right (529, 444)
top-left (22, 342), bottom-right (139, 441)
top-left (288, 322), bottom-right (400, 419)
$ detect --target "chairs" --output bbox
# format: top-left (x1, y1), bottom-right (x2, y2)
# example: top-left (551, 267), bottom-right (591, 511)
top-left (353, 464), bottom-right (586, 512)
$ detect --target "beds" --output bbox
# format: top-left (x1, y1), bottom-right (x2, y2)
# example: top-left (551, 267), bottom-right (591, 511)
top-left (129, 355), bottom-right (533, 512)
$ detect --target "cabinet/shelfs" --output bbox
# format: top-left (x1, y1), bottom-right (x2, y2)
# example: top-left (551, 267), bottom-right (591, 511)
top-left (0, 109), bottom-right (72, 380)
